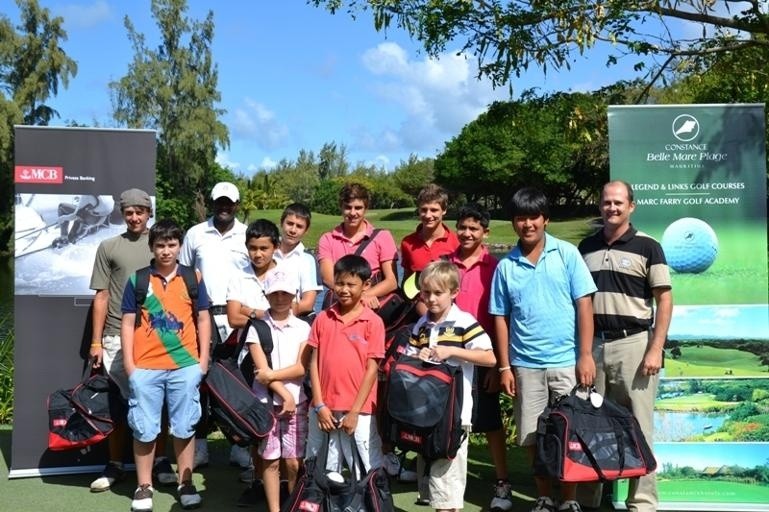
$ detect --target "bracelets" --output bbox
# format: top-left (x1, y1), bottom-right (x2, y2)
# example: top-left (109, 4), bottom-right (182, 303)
top-left (497, 366), bottom-right (511, 373)
top-left (90, 343), bottom-right (105, 347)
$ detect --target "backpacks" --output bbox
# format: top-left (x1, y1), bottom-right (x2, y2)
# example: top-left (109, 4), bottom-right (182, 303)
top-left (377, 292), bottom-right (467, 460)
top-left (204, 319), bottom-right (275, 447)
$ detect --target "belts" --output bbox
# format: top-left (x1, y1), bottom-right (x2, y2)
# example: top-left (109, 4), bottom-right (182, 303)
top-left (209, 306), bottom-right (227, 314)
top-left (595, 329), bottom-right (646, 342)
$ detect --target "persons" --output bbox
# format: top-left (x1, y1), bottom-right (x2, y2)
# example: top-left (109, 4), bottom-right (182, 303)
top-left (121, 218), bottom-right (214, 511)
top-left (51, 194), bottom-right (116, 244)
top-left (488, 185), bottom-right (600, 512)
top-left (577, 182), bottom-right (674, 512)
top-left (88, 188), bottom-right (177, 492)
top-left (179, 180), bottom-right (517, 511)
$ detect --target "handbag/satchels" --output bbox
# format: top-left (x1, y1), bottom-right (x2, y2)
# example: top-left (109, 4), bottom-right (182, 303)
top-left (47, 358), bottom-right (117, 451)
top-left (532, 383), bottom-right (656, 482)
top-left (282, 425), bottom-right (394, 512)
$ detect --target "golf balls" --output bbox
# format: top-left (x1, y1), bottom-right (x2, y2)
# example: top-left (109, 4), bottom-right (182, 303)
top-left (661, 218), bottom-right (718, 274)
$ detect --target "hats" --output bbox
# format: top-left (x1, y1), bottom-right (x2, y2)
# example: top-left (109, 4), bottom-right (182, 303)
top-left (210, 181), bottom-right (240, 203)
top-left (120, 188), bottom-right (152, 210)
top-left (263, 268), bottom-right (299, 296)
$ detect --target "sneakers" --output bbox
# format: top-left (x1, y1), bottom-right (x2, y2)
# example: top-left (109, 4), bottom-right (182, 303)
top-left (382, 453), bottom-right (417, 481)
top-left (490, 480), bottom-right (512, 511)
top-left (530, 497), bottom-right (583, 512)
top-left (229, 445), bottom-right (266, 506)
top-left (91, 441), bottom-right (208, 511)
